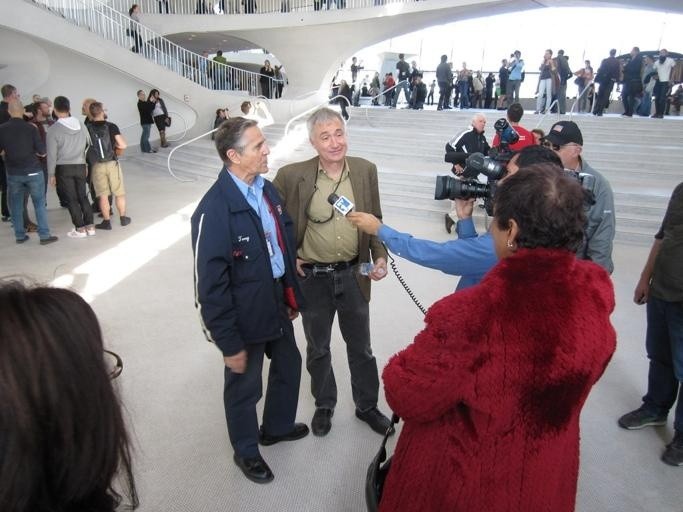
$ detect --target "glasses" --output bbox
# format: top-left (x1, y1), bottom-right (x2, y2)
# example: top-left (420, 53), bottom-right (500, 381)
top-left (543, 142), bottom-right (569, 150)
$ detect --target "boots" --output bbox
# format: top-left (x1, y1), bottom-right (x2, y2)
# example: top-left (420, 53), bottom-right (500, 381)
top-left (160, 133), bottom-right (170, 147)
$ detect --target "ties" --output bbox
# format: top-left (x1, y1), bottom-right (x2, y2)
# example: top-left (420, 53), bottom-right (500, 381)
top-left (267, 67), bottom-right (270, 72)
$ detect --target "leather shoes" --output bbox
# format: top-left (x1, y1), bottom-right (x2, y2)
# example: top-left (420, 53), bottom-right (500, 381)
top-left (355, 405), bottom-right (395, 436)
top-left (259, 423), bottom-right (310, 446)
top-left (119, 217), bottom-right (131, 226)
top-left (39, 236), bottom-right (58, 245)
top-left (98, 209), bottom-right (113, 217)
top-left (657, 114), bottom-right (663, 118)
top-left (311, 406), bottom-right (334, 437)
top-left (592, 112), bottom-right (603, 116)
top-left (141, 149), bottom-right (157, 153)
top-left (234, 452), bottom-right (274, 483)
top-left (95, 223), bottom-right (112, 230)
top-left (16, 235), bottom-right (29, 243)
top-left (651, 113), bottom-right (656, 118)
top-left (90, 208), bottom-right (100, 212)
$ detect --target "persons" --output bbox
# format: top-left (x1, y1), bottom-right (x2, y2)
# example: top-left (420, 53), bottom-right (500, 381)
top-left (619, 182), bottom-right (682, 467)
top-left (374, 161), bottom-right (619, 512)
top-left (128, 4), bottom-right (142, 52)
top-left (240, 101), bottom-right (275, 130)
top-left (531, 129), bottom-right (544, 146)
top-left (190, 115), bottom-right (311, 483)
top-left (539, 122), bottom-right (618, 275)
top-left (211, 108), bottom-right (229, 140)
top-left (535, 49), bottom-right (572, 114)
top-left (436, 50), bottom-right (524, 111)
top-left (337, 80), bottom-right (351, 120)
top-left (576, 46), bottom-right (683, 121)
top-left (271, 107), bottom-right (398, 438)
top-left (154, 0), bottom-right (432, 15)
top-left (136, 89), bottom-right (159, 153)
top-left (345, 145), bottom-right (563, 295)
top-left (492, 104), bottom-right (535, 150)
top-left (329, 54), bottom-right (435, 109)
top-left (147, 89), bottom-right (171, 147)
top-left (0, 82), bottom-right (130, 246)
top-left (444, 112), bottom-right (490, 234)
top-left (0, 277), bottom-right (141, 511)
top-left (197, 50), bottom-right (285, 100)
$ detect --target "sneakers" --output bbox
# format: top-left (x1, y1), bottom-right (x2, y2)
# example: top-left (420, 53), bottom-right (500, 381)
top-left (622, 112), bottom-right (626, 115)
top-left (661, 435), bottom-right (683, 466)
top-left (370, 102), bottom-right (396, 107)
top-left (85, 223), bottom-right (96, 235)
top-left (67, 226), bottom-right (87, 238)
top-left (437, 106), bottom-right (507, 111)
top-left (626, 113), bottom-right (632, 116)
top-left (1, 216), bottom-right (12, 222)
top-left (24, 222), bottom-right (39, 231)
top-left (618, 407), bottom-right (667, 430)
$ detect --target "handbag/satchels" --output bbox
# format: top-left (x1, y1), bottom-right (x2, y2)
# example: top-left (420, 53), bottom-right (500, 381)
top-left (520, 71), bottom-right (525, 82)
top-left (574, 76), bottom-right (585, 85)
top-left (165, 116), bottom-right (171, 127)
top-left (364, 446), bottom-right (392, 511)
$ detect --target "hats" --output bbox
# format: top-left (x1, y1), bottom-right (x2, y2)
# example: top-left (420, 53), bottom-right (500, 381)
top-left (539, 121), bottom-right (583, 145)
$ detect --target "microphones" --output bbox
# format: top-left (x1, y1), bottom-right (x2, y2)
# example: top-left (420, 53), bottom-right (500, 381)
top-left (328, 193), bottom-right (353, 216)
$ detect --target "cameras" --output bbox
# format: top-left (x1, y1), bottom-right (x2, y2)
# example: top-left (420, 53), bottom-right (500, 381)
top-left (511, 54), bottom-right (514, 57)
top-left (222, 108), bottom-right (229, 113)
